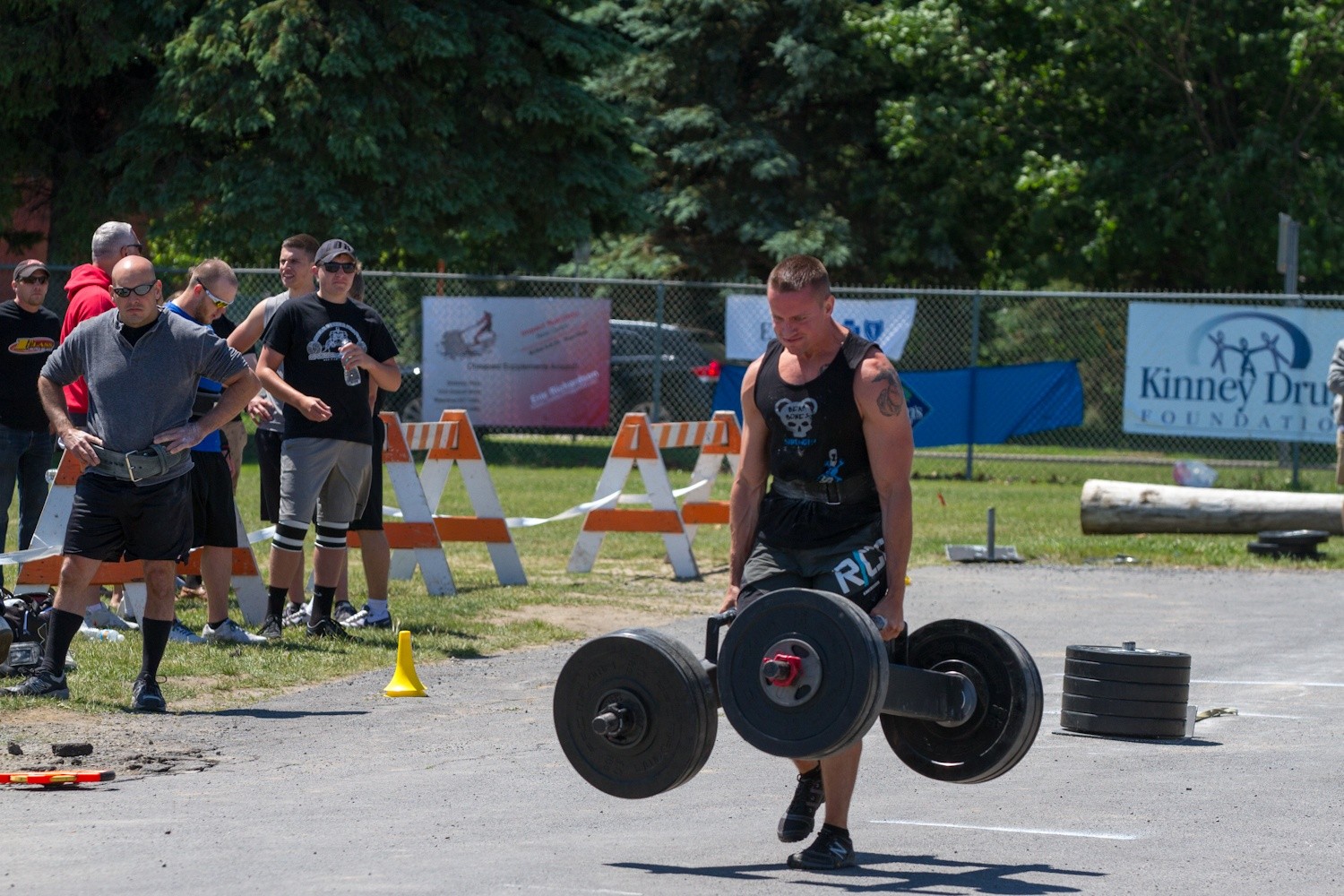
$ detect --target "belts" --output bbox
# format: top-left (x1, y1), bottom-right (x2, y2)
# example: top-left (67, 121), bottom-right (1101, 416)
top-left (87, 442), bottom-right (191, 483)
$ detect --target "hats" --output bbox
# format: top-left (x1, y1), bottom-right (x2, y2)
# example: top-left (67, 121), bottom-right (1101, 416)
top-left (314, 239), bottom-right (356, 265)
top-left (13, 258), bottom-right (50, 281)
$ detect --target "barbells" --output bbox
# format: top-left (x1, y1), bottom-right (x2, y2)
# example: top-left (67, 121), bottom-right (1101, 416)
top-left (715, 586), bottom-right (1047, 789)
top-left (547, 610), bottom-right (721, 805)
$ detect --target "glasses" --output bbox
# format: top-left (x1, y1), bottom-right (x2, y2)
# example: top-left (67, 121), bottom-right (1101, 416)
top-left (113, 280), bottom-right (157, 298)
top-left (196, 277), bottom-right (234, 309)
top-left (16, 275), bottom-right (47, 284)
top-left (316, 261), bottom-right (355, 274)
top-left (121, 243), bottom-right (143, 254)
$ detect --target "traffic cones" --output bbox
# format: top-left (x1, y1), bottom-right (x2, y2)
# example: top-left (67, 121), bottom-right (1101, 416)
top-left (380, 629), bottom-right (428, 698)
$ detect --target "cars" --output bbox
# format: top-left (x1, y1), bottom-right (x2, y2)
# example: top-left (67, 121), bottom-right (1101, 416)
top-left (377, 312), bottom-right (751, 453)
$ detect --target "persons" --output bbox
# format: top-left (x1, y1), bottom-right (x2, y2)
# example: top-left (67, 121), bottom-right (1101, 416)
top-left (717, 254), bottom-right (914, 871)
top-left (1327, 338), bottom-right (1344, 486)
top-left (0, 221), bottom-right (402, 646)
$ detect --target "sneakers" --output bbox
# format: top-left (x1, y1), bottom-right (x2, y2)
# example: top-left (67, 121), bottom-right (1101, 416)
top-left (283, 601), bottom-right (298, 618)
top-left (256, 614), bottom-right (282, 639)
top-left (787, 831), bottom-right (855, 869)
top-left (339, 604), bottom-right (392, 628)
top-left (306, 615), bottom-right (364, 643)
top-left (778, 771), bottom-right (826, 842)
top-left (333, 601), bottom-right (358, 622)
top-left (181, 586), bottom-right (208, 601)
top-left (130, 674), bottom-right (167, 711)
top-left (84, 601), bottom-right (139, 631)
top-left (201, 619), bottom-right (266, 646)
top-left (168, 623), bottom-right (209, 645)
top-left (2, 667), bottom-right (69, 699)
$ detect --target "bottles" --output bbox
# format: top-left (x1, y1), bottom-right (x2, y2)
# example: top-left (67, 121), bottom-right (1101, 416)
top-left (84, 628), bottom-right (124, 643)
top-left (339, 339), bottom-right (361, 387)
top-left (45, 469), bottom-right (57, 482)
